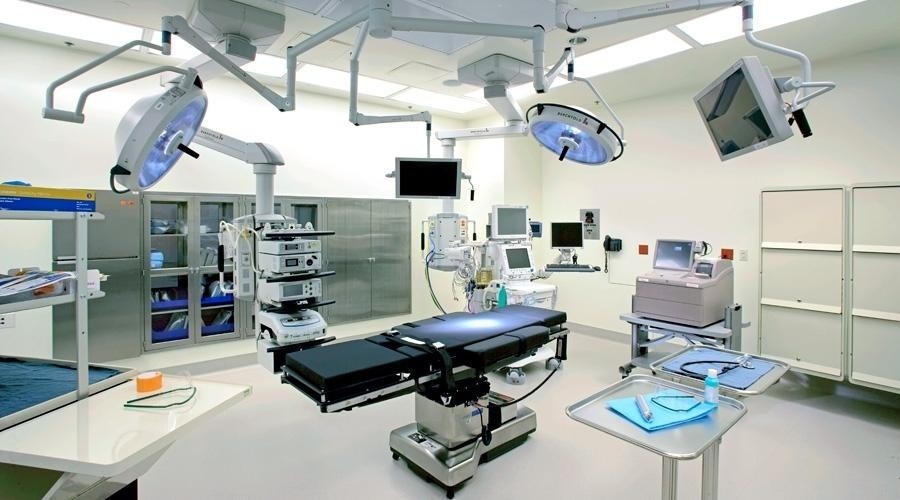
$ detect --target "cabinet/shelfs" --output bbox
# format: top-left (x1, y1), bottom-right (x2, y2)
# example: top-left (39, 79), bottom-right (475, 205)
top-left (53, 189), bottom-right (412, 366)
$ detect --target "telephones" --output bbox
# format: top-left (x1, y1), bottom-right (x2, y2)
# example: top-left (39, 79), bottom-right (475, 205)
top-left (603, 235), bottom-right (622, 273)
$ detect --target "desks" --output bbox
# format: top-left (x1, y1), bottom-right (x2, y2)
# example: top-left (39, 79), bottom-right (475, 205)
top-left (565, 375), bottom-right (746, 500)
top-left (0, 372), bottom-right (250, 500)
top-left (651, 342), bottom-right (790, 402)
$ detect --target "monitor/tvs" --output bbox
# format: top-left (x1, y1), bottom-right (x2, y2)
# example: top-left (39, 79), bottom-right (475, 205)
top-left (501, 244), bottom-right (536, 275)
top-left (653, 238), bottom-right (696, 272)
top-left (492, 204), bottom-right (529, 238)
top-left (395, 158), bottom-right (462, 199)
top-left (550, 221), bottom-right (584, 249)
top-left (693, 56), bottom-right (795, 162)
